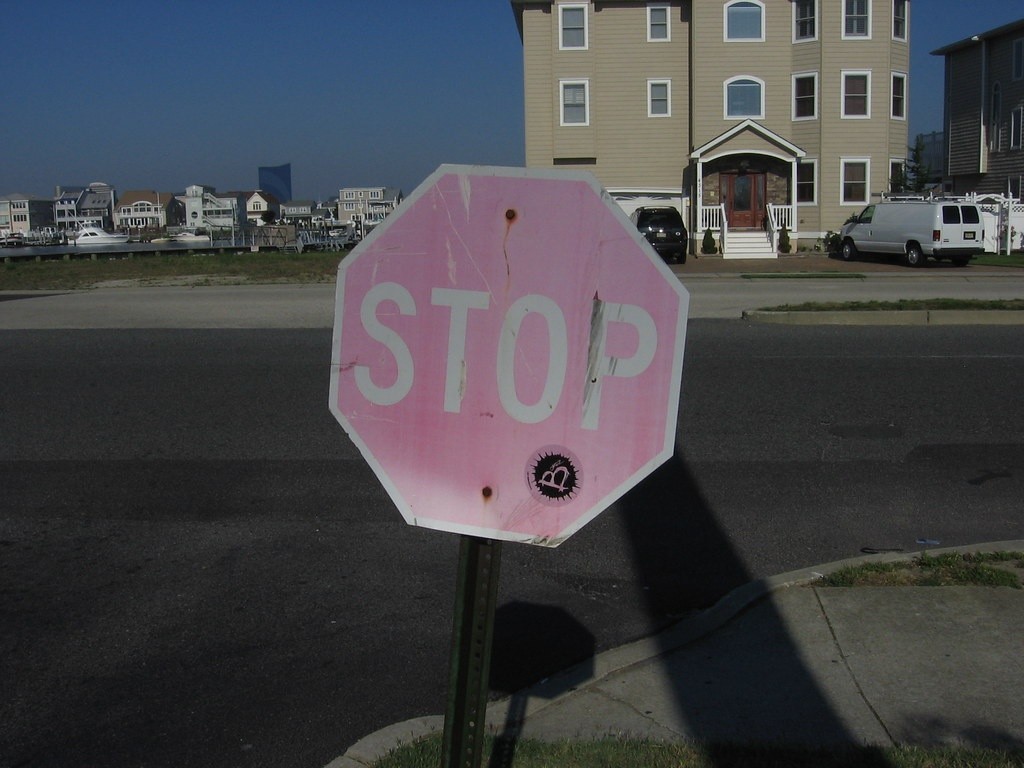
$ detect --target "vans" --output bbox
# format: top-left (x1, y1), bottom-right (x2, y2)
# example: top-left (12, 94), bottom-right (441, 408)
top-left (838, 198), bottom-right (986, 267)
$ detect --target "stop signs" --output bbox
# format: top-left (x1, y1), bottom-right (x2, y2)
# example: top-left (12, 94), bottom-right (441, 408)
top-left (325, 162), bottom-right (691, 550)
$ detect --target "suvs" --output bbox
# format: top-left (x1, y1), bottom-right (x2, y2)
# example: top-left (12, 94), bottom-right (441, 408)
top-left (627, 205), bottom-right (688, 266)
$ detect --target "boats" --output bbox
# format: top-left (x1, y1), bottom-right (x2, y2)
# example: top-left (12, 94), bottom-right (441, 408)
top-left (59, 226), bottom-right (131, 244)
top-left (151, 231), bottom-right (210, 245)
